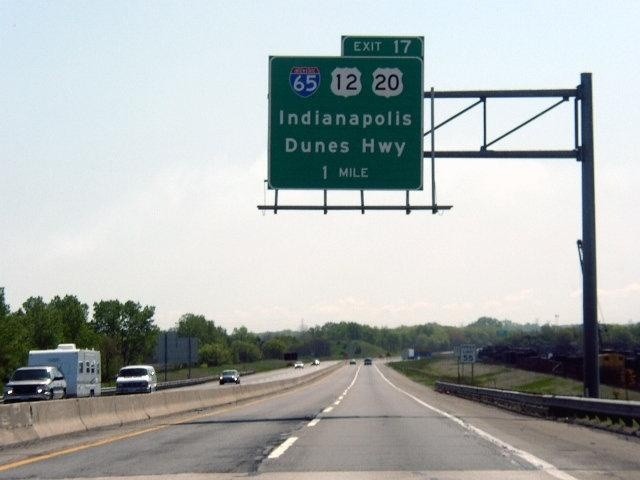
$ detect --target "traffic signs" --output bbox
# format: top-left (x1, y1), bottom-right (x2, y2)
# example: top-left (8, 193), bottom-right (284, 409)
top-left (460, 344), bottom-right (476, 363)
top-left (266, 34), bottom-right (424, 190)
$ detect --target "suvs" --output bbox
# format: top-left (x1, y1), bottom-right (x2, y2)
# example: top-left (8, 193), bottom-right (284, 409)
top-left (4, 365), bottom-right (68, 403)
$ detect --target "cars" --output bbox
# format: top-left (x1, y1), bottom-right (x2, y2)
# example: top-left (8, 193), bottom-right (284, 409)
top-left (311, 360), bottom-right (320, 366)
top-left (350, 359), bottom-right (356, 365)
top-left (219, 370), bottom-right (241, 385)
top-left (294, 360), bottom-right (304, 369)
top-left (364, 359), bottom-right (372, 365)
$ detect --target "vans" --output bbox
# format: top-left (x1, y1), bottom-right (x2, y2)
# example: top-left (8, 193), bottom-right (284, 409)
top-left (115, 365), bottom-right (157, 394)
